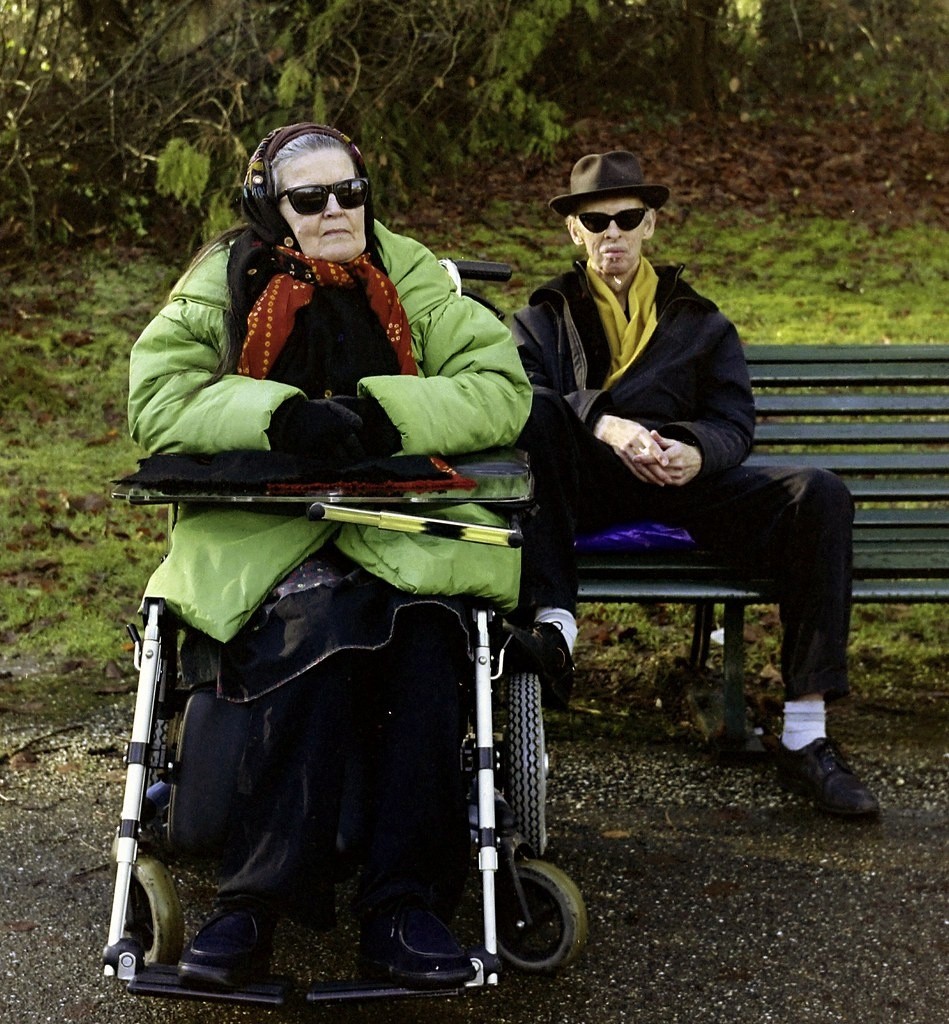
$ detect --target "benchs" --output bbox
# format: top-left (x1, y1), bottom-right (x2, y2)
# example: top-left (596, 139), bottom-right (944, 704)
top-left (576, 343), bottom-right (949, 741)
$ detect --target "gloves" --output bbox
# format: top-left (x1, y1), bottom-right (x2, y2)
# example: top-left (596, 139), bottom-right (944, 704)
top-left (331, 391), bottom-right (401, 459)
top-left (268, 397), bottom-right (365, 469)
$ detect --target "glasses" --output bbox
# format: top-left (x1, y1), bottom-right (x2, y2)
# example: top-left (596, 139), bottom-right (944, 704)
top-left (575, 205), bottom-right (652, 232)
top-left (275, 176), bottom-right (370, 215)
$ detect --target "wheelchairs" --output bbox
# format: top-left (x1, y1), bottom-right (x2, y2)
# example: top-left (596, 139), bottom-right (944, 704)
top-left (103, 259), bottom-right (593, 1005)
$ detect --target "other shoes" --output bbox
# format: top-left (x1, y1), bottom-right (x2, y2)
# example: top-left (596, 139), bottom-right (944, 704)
top-left (176, 902), bottom-right (274, 993)
top-left (360, 901), bottom-right (474, 988)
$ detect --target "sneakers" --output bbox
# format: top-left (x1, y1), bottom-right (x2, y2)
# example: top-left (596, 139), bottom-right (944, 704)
top-left (772, 736), bottom-right (881, 821)
top-left (495, 619), bottom-right (576, 712)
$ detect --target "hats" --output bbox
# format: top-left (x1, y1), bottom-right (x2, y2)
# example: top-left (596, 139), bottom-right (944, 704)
top-left (549, 150), bottom-right (671, 214)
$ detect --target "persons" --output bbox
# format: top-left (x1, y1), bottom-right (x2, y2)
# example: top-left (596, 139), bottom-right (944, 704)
top-left (503, 150), bottom-right (882, 815)
top-left (127, 122), bottom-right (533, 988)
